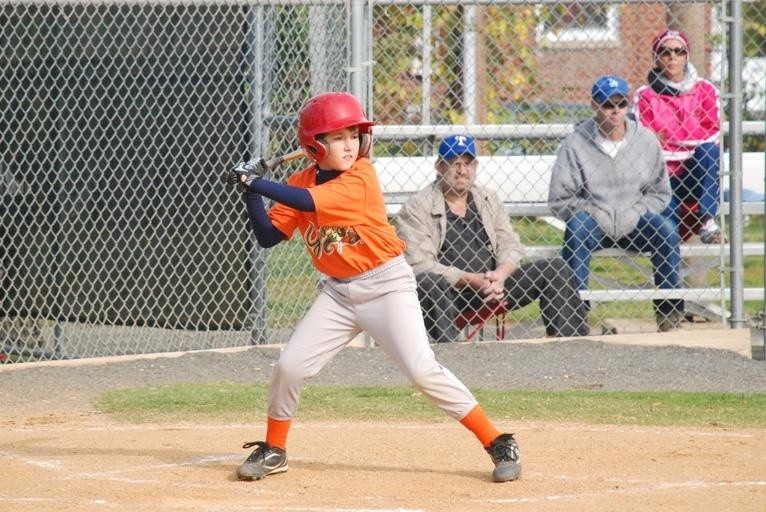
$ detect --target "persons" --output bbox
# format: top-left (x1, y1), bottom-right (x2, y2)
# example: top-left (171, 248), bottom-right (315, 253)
top-left (632, 31), bottom-right (728, 245)
top-left (390, 133), bottom-right (589, 343)
top-left (548, 74), bottom-right (686, 332)
top-left (233, 91), bottom-right (523, 483)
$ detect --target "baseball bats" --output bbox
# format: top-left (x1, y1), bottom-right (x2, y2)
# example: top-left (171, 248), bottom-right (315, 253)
top-left (225, 149), bottom-right (305, 185)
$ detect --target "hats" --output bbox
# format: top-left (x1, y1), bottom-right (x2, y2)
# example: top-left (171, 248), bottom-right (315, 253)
top-left (591, 75), bottom-right (628, 105)
top-left (651, 30), bottom-right (692, 56)
top-left (439, 135), bottom-right (477, 160)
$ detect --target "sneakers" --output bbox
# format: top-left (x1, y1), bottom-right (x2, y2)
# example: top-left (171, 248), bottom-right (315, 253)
top-left (699, 220), bottom-right (726, 243)
top-left (238, 441), bottom-right (287, 481)
top-left (485, 433), bottom-right (522, 483)
top-left (658, 310), bottom-right (707, 333)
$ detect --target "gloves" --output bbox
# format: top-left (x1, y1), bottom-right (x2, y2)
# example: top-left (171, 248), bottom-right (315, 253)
top-left (231, 156), bottom-right (268, 191)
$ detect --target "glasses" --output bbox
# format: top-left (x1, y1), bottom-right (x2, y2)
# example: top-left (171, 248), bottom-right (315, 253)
top-left (658, 46), bottom-right (687, 57)
top-left (603, 101), bottom-right (628, 109)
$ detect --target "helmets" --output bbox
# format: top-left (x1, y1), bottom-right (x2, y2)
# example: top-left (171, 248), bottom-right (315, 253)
top-left (299, 91), bottom-right (377, 164)
